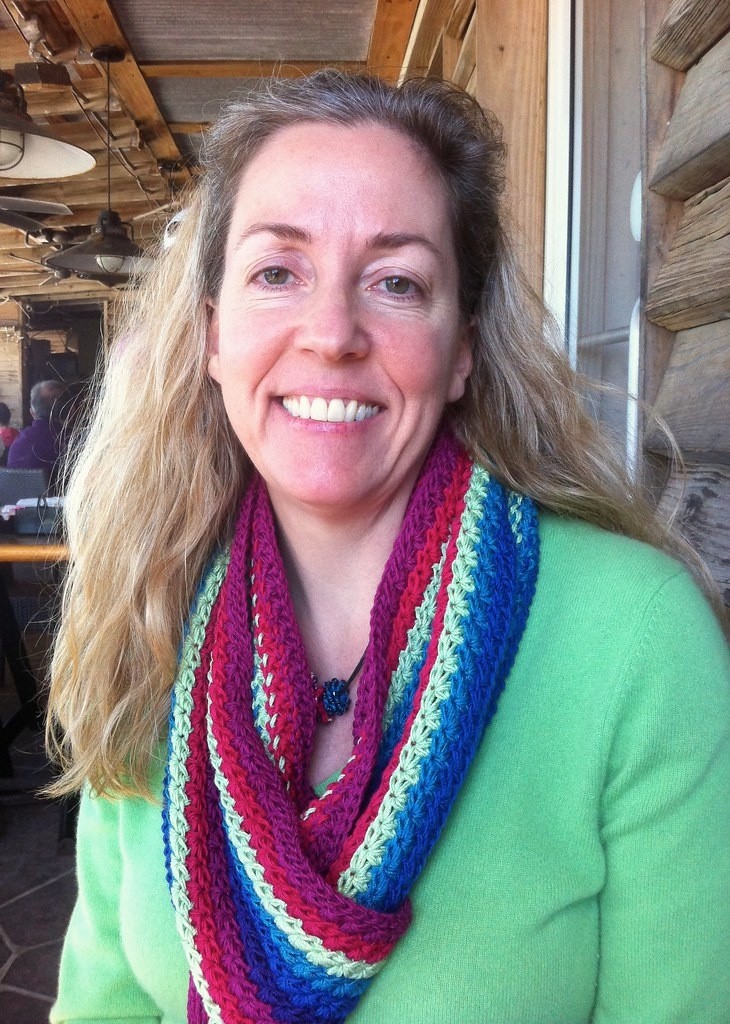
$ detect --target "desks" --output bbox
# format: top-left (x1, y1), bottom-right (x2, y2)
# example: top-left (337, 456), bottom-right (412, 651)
top-left (0, 535), bottom-right (70, 781)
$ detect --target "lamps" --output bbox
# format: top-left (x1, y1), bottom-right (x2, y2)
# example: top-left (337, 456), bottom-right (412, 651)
top-left (0, 64), bottom-right (97, 182)
top-left (45, 43), bottom-right (156, 275)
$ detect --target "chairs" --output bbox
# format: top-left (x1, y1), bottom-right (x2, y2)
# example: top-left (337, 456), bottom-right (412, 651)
top-left (0, 466), bottom-right (66, 587)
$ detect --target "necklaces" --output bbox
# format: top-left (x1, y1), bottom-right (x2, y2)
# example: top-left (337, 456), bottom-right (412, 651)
top-left (310, 641), bottom-right (370, 725)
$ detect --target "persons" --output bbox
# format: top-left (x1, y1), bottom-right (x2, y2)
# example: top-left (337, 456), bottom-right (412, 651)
top-left (21, 50), bottom-right (730, 1024)
top-left (0, 402), bottom-right (20, 449)
top-left (7, 381), bottom-right (75, 495)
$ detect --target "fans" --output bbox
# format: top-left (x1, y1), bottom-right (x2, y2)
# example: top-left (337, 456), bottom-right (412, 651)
top-left (0, 193), bottom-right (73, 237)
top-left (9, 231), bottom-right (114, 286)
